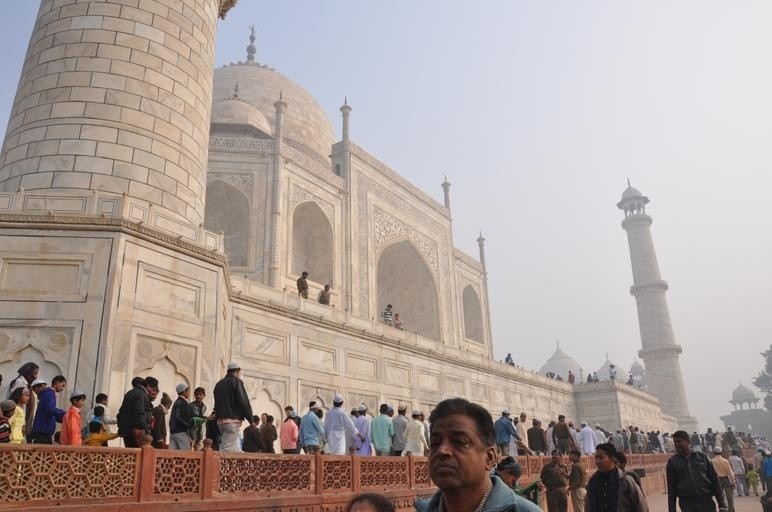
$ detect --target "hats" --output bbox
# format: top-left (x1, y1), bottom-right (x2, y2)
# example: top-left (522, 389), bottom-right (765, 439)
top-left (502, 408), bottom-right (511, 414)
top-left (161, 392), bottom-right (171, 404)
top-left (352, 405), bottom-right (366, 412)
top-left (31, 379), bottom-right (47, 387)
top-left (1, 400), bottom-right (16, 411)
top-left (580, 421), bottom-right (600, 427)
top-left (758, 447), bottom-right (762, 450)
top-left (412, 409), bottom-right (425, 416)
top-left (290, 412), bottom-right (297, 418)
top-left (228, 364), bottom-right (241, 369)
top-left (334, 395), bottom-right (343, 403)
top-left (70, 391), bottom-right (84, 399)
top-left (176, 384), bottom-right (188, 393)
top-left (398, 405), bottom-right (406, 411)
top-left (714, 447), bottom-right (722, 453)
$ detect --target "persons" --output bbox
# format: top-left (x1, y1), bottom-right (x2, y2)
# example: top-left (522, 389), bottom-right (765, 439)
top-left (0, 362), bottom-right (431, 456)
top-left (318, 285), bottom-right (330, 305)
top-left (347, 397), bottom-right (771, 511)
top-left (297, 272), bottom-right (309, 299)
top-left (394, 314), bottom-right (404, 330)
top-left (381, 304), bottom-right (393, 327)
